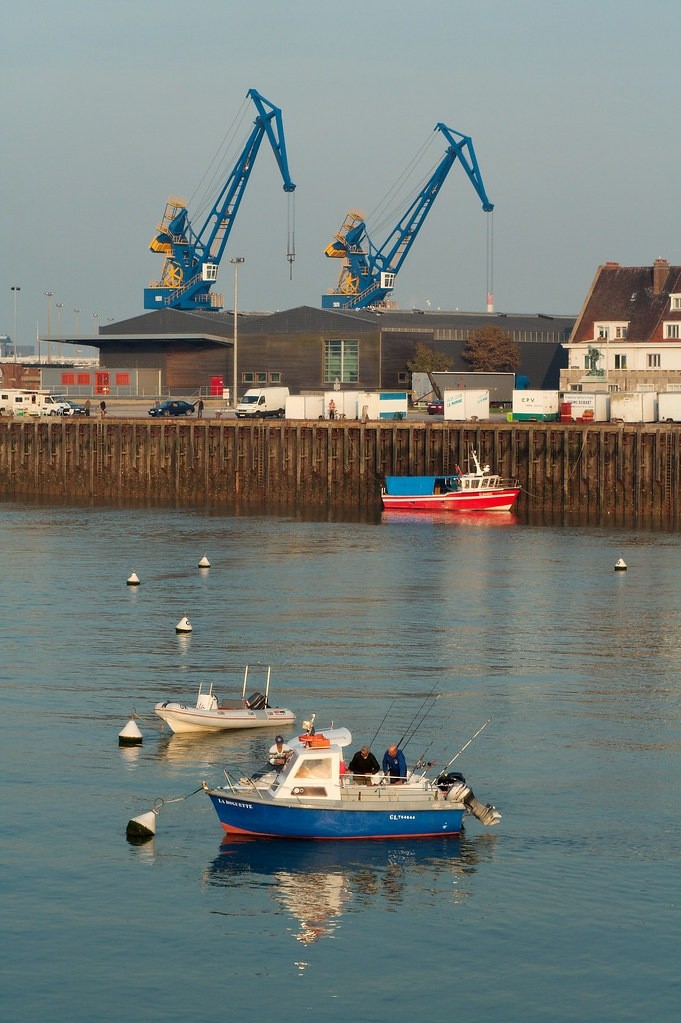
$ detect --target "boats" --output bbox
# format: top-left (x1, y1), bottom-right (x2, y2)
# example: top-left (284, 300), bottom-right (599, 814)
top-left (154, 665), bottom-right (297, 735)
top-left (381, 449), bottom-right (522, 508)
top-left (200, 680), bottom-right (503, 840)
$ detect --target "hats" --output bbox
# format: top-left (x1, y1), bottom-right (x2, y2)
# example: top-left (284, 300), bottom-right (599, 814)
top-left (275, 735), bottom-right (284, 743)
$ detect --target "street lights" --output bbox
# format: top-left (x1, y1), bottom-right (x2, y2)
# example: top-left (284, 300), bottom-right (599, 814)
top-left (11, 284), bottom-right (21, 363)
top-left (55, 303), bottom-right (64, 358)
top-left (73, 308), bottom-right (82, 358)
top-left (91, 313), bottom-right (100, 336)
top-left (45, 292), bottom-right (56, 363)
top-left (229, 256), bottom-right (245, 409)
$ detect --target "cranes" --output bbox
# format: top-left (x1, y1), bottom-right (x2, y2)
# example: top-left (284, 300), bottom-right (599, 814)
top-left (143, 88), bottom-right (296, 312)
top-left (321, 123), bottom-right (494, 309)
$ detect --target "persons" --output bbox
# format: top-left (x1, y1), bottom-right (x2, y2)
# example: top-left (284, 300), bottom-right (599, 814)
top-left (268, 735), bottom-right (293, 777)
top-left (325, 759), bottom-right (346, 779)
top-left (348, 746), bottom-right (380, 786)
top-left (329, 399), bottom-right (335, 419)
top-left (100, 401), bottom-right (106, 415)
top-left (199, 398), bottom-right (204, 418)
top-left (382, 745), bottom-right (407, 785)
top-left (85, 398), bottom-right (91, 416)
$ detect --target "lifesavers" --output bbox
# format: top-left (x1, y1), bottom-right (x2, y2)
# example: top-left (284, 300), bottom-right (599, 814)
top-left (298, 734), bottom-right (323, 741)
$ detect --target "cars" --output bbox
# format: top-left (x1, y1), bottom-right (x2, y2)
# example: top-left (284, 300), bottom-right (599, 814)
top-left (66, 400), bottom-right (86, 416)
top-left (147, 400), bottom-right (195, 417)
top-left (427, 400), bottom-right (444, 415)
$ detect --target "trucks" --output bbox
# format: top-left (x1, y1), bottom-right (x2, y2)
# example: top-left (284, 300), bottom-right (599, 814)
top-left (0, 388), bottom-right (74, 416)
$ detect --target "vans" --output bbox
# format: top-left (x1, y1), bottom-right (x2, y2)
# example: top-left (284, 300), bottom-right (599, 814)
top-left (235, 386), bottom-right (290, 419)
